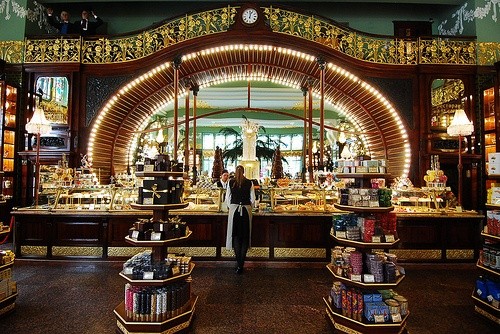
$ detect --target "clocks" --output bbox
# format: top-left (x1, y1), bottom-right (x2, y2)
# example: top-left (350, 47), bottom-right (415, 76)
top-left (242, 8), bottom-right (258, 24)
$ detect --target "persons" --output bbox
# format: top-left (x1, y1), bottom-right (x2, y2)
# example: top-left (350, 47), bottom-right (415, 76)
top-left (46, 7), bottom-right (73, 34)
top-left (212, 170), bottom-right (229, 190)
top-left (74, 10), bottom-right (104, 35)
top-left (225, 165), bottom-right (256, 275)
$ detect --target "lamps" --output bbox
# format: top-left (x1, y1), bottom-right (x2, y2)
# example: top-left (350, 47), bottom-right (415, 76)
top-left (448, 109), bottom-right (475, 207)
top-left (25, 110), bottom-right (53, 209)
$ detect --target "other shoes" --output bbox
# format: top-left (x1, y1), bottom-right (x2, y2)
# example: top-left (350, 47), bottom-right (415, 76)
top-left (237, 267), bottom-right (243, 274)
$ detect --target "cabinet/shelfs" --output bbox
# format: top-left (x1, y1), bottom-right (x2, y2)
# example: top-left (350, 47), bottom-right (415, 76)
top-left (0, 200), bottom-right (21, 316)
top-left (471, 174), bottom-right (500, 326)
top-left (322, 174), bottom-right (410, 334)
top-left (114, 171), bottom-right (200, 334)
